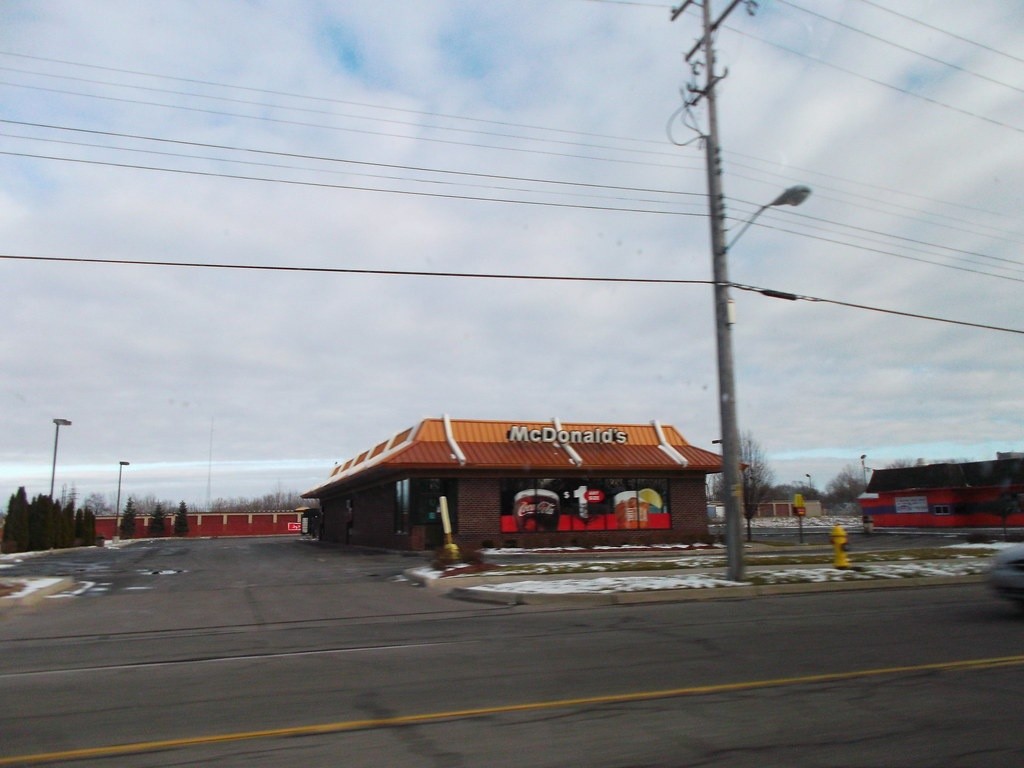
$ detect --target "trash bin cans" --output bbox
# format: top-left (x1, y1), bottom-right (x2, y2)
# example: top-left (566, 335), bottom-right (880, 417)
top-left (112, 536), bottom-right (121, 544)
top-left (863, 515), bottom-right (874, 534)
top-left (97, 537), bottom-right (104, 547)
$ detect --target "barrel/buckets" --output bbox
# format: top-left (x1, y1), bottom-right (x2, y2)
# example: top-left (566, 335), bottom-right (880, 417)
top-left (112, 535), bottom-right (119, 544)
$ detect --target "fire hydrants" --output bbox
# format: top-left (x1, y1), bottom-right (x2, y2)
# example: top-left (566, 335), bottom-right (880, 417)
top-left (831, 522), bottom-right (852, 571)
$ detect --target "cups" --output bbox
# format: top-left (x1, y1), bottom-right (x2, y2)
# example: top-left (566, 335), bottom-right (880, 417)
top-left (514, 489), bottom-right (560, 532)
top-left (613, 490), bottom-right (649, 529)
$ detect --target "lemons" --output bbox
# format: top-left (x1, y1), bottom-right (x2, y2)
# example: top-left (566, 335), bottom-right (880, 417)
top-left (638, 489), bottom-right (662, 509)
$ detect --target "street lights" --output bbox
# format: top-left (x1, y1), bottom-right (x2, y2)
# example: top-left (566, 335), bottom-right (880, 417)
top-left (50, 418), bottom-right (72, 496)
top-left (806, 473), bottom-right (812, 494)
top-left (712, 182), bottom-right (813, 576)
top-left (860, 454), bottom-right (869, 488)
top-left (115, 461), bottom-right (130, 535)
